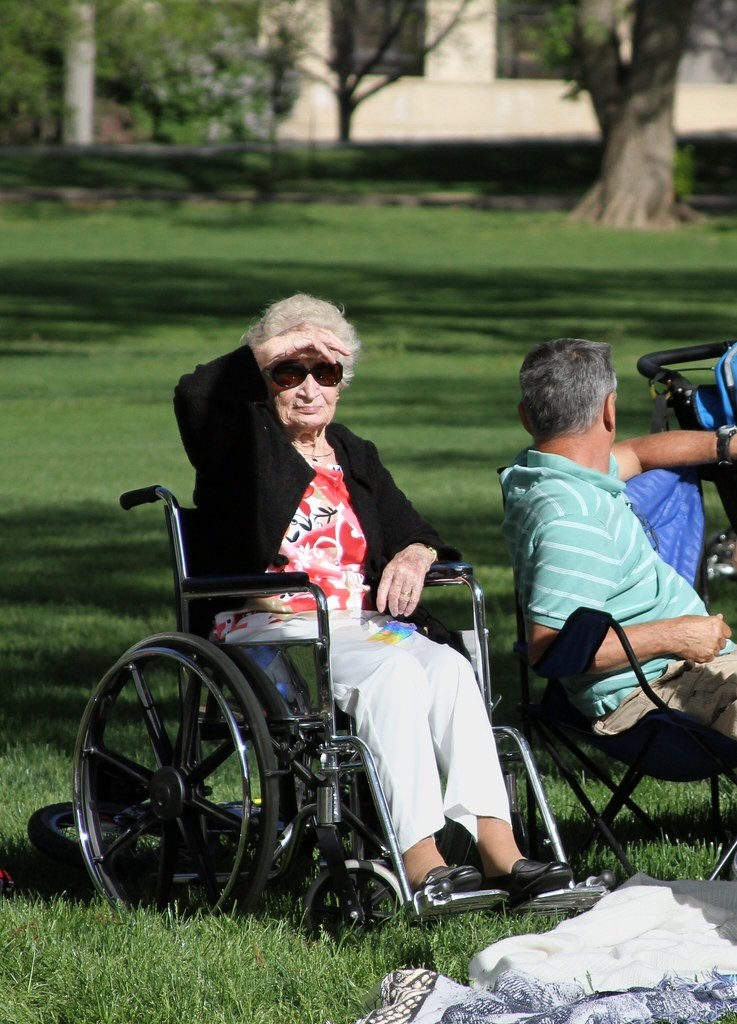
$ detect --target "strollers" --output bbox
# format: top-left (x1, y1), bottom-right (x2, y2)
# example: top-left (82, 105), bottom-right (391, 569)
top-left (635, 338), bottom-right (736, 593)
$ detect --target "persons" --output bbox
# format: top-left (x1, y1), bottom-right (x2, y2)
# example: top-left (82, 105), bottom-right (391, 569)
top-left (171, 290), bottom-right (577, 920)
top-left (495, 334), bottom-right (737, 763)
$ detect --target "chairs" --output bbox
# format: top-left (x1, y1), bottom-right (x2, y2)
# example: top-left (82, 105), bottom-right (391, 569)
top-left (498, 465), bottom-right (737, 881)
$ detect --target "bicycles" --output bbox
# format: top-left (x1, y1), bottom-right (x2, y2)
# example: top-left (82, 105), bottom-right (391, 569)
top-left (24, 796), bottom-right (312, 882)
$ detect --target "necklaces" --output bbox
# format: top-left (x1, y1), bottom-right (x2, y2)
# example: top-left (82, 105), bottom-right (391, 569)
top-left (293, 446), bottom-right (335, 463)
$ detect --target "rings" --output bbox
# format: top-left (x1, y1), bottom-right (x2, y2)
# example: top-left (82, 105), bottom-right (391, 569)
top-left (400, 591), bottom-right (413, 598)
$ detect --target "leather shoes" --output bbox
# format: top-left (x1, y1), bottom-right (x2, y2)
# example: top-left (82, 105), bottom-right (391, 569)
top-left (484, 859), bottom-right (576, 914)
top-left (415, 863), bottom-right (484, 895)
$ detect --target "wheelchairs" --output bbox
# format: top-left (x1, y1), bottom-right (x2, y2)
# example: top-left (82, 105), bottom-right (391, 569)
top-left (75, 479), bottom-right (614, 957)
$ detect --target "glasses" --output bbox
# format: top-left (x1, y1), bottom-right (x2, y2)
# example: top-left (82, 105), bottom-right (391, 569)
top-left (261, 359), bottom-right (344, 389)
